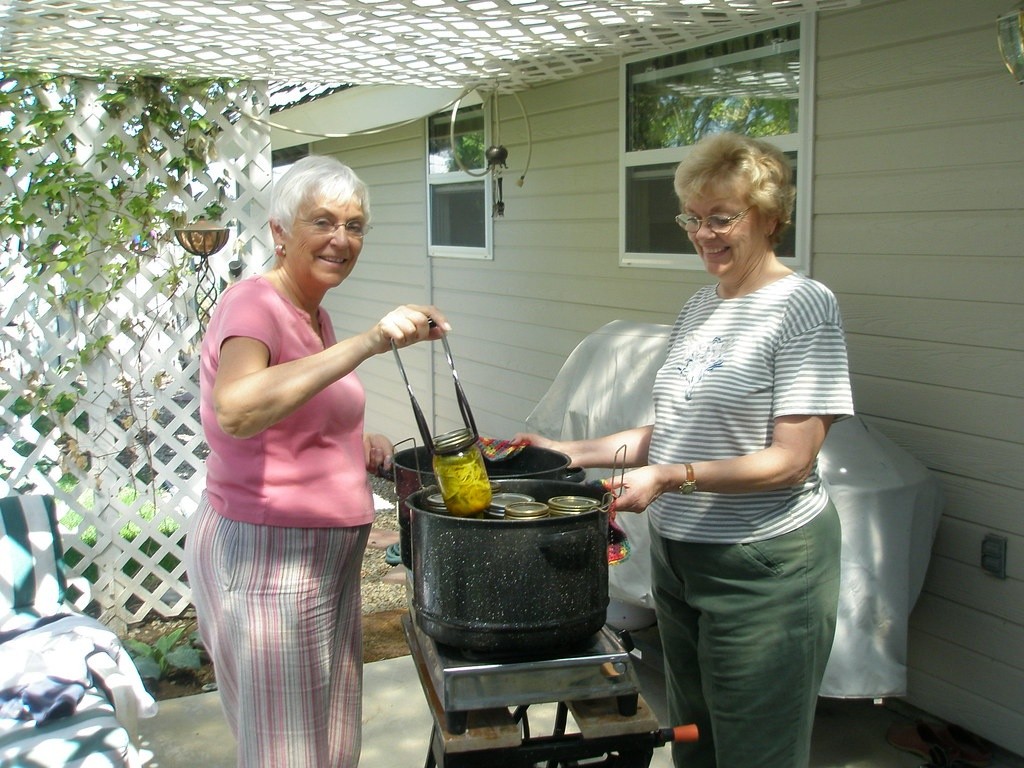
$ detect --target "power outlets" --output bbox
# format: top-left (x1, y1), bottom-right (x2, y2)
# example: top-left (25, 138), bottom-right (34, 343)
top-left (980, 532), bottom-right (1006, 579)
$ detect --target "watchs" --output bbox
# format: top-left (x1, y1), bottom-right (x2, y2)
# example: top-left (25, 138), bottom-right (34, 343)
top-left (679, 463), bottom-right (697, 494)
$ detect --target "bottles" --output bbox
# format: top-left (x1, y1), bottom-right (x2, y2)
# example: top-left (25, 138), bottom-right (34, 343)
top-left (431, 428), bottom-right (601, 523)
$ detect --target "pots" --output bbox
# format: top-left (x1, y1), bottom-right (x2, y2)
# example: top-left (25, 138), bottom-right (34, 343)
top-left (389, 439), bottom-right (626, 650)
top-left (378, 437), bottom-right (585, 571)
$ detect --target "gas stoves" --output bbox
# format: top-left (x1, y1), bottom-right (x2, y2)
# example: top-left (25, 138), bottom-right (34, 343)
top-left (397, 569), bottom-right (642, 733)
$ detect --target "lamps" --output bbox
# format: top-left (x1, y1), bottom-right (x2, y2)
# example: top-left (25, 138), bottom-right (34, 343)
top-left (996, 0), bottom-right (1024, 85)
top-left (175, 216), bottom-right (230, 340)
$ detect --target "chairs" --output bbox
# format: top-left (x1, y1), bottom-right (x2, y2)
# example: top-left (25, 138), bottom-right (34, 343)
top-left (0, 494), bottom-right (157, 768)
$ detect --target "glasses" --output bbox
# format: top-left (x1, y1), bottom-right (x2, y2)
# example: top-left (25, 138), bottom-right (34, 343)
top-left (295, 218), bottom-right (373, 237)
top-left (675, 203), bottom-right (755, 234)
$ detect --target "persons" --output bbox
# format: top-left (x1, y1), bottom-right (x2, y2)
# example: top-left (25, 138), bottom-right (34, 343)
top-left (184, 155), bottom-right (452, 768)
top-left (513, 131), bottom-right (855, 768)
